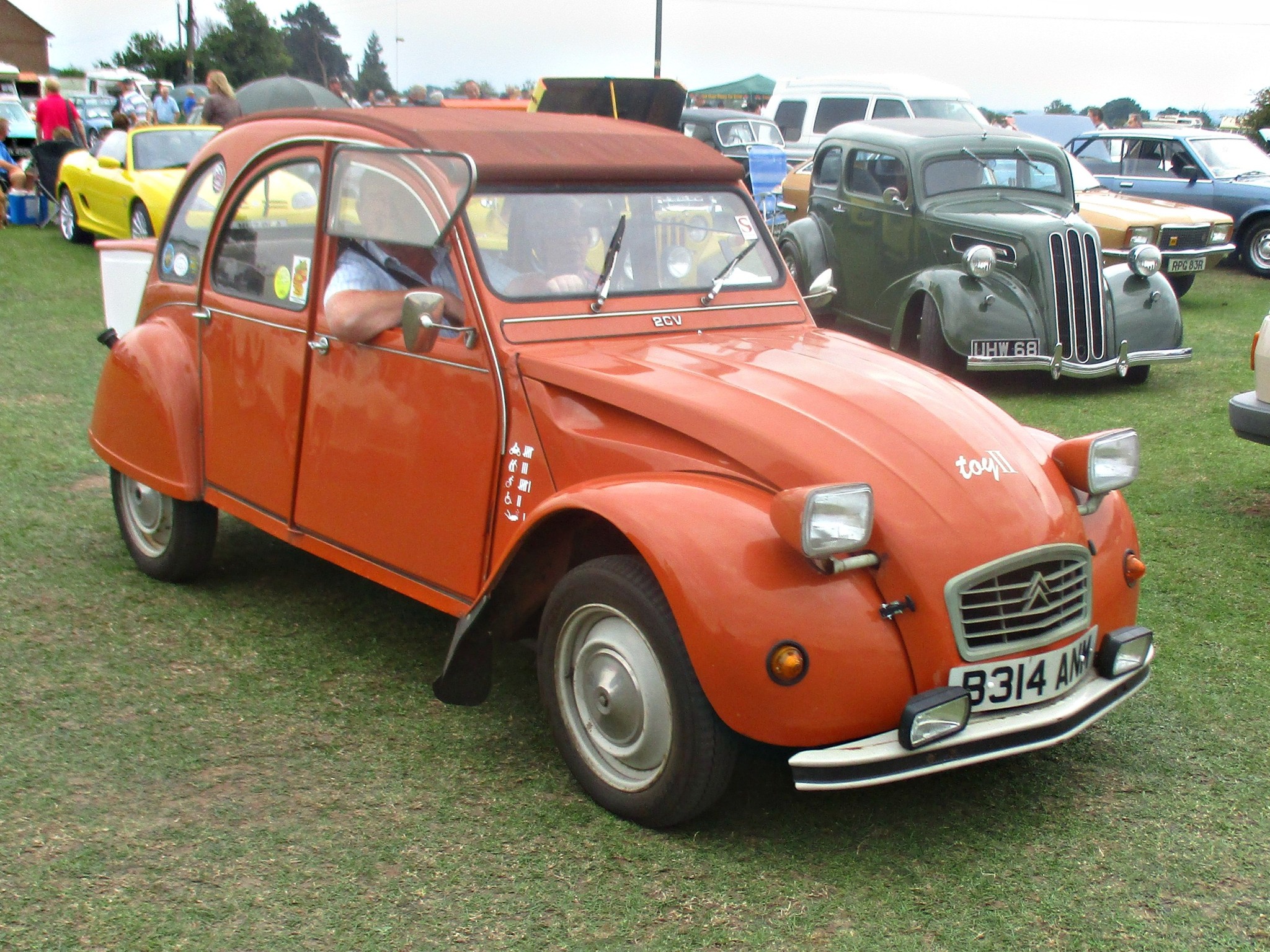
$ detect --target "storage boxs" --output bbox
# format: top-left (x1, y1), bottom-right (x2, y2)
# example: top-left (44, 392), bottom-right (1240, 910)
top-left (7, 193), bottom-right (49, 225)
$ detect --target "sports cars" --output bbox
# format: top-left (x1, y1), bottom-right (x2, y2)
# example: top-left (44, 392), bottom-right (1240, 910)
top-left (56, 123), bottom-right (318, 246)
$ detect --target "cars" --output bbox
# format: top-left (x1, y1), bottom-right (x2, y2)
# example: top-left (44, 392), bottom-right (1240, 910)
top-left (1013, 112), bottom-right (1270, 279)
top-left (1228, 311), bottom-right (1270, 447)
top-left (775, 116), bottom-right (1195, 386)
top-left (87, 108), bottom-right (1160, 832)
top-left (980, 132), bottom-right (1236, 300)
top-left (338, 77), bottom-right (993, 289)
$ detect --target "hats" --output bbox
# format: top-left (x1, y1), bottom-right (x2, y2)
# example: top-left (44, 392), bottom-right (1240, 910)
top-left (373, 89), bottom-right (387, 102)
top-left (122, 79), bottom-right (133, 85)
top-left (329, 77), bottom-right (341, 83)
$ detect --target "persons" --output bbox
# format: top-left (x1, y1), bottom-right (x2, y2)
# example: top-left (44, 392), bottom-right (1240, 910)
top-left (1125, 114), bottom-right (1143, 148)
top-left (151, 82), bottom-right (180, 125)
top-left (749, 101), bottom-right (762, 116)
top-left (1086, 107), bottom-right (1110, 147)
top-left (36, 79), bottom-right (90, 149)
top-left (322, 169), bottom-right (585, 343)
top-left (362, 89), bottom-right (391, 107)
top-left (462, 79), bottom-right (483, 99)
top-left (328, 77), bottom-right (351, 108)
top-left (118, 76), bottom-right (151, 128)
top-left (1, 119), bottom-right (36, 193)
top-left (202, 71), bottom-right (242, 126)
top-left (403, 86), bottom-right (428, 106)
top-left (181, 89), bottom-right (205, 121)
top-left (530, 198), bottom-right (623, 292)
top-left (112, 113), bottom-right (131, 132)
top-left (52, 126), bottom-right (74, 142)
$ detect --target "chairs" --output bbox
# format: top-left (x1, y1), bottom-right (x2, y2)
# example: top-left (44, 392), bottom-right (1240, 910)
top-left (749, 145), bottom-right (788, 236)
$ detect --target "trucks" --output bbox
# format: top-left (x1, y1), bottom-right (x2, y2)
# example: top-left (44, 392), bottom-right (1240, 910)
top-left (0, 61), bottom-right (175, 149)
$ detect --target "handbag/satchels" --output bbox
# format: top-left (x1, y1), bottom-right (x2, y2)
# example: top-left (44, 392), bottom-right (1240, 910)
top-left (71, 125), bottom-right (87, 149)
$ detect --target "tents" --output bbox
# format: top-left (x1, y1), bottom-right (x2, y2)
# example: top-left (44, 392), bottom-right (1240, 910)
top-left (685, 74), bottom-right (776, 109)
